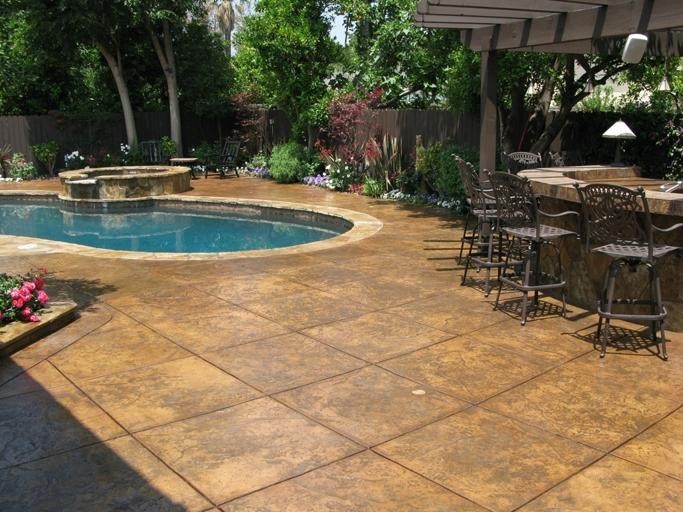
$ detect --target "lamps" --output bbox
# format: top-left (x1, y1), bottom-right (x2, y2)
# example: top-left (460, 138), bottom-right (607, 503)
top-left (622, 33), bottom-right (648, 64)
top-left (601, 54), bottom-right (640, 142)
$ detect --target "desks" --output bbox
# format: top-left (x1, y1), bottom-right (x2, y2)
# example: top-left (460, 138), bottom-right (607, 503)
top-left (169, 158), bottom-right (199, 178)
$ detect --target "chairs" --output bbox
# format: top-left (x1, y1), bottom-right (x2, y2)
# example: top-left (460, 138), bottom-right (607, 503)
top-left (140, 140), bottom-right (163, 165)
top-left (450, 151), bottom-right (579, 326)
top-left (204, 138), bottom-right (242, 179)
top-left (573, 180), bottom-right (683, 361)
